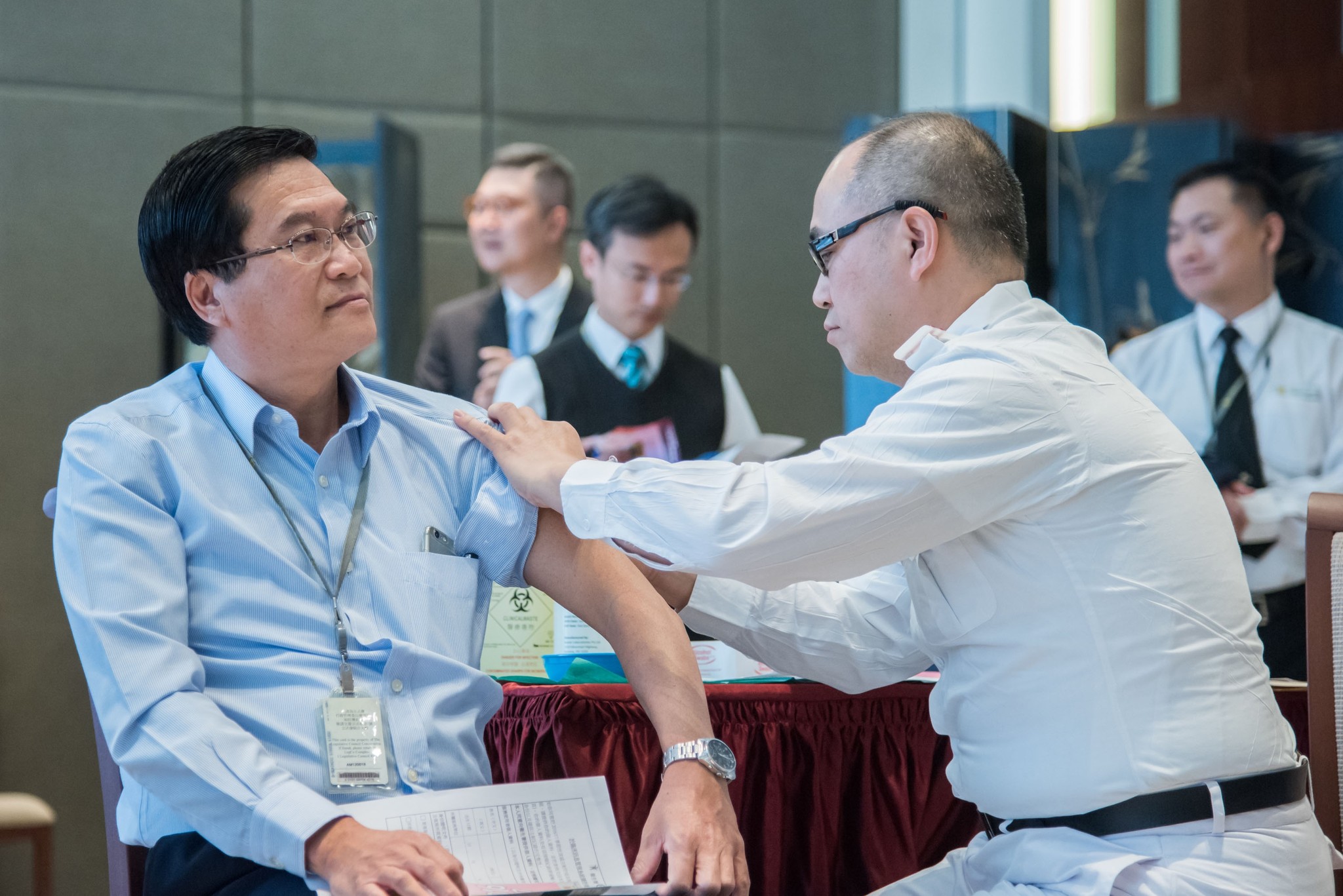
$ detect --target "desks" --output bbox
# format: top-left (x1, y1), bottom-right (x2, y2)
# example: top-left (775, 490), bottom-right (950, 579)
top-left (486, 676), bottom-right (983, 896)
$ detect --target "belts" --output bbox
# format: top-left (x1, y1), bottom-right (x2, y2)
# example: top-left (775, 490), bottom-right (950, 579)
top-left (1252, 591), bottom-right (1304, 627)
top-left (976, 750), bottom-right (1310, 840)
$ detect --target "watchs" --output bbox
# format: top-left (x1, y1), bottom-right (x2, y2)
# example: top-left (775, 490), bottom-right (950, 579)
top-left (661, 738), bottom-right (737, 785)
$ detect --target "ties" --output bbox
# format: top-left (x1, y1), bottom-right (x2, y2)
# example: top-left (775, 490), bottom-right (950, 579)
top-left (1210, 328), bottom-right (1280, 562)
top-left (620, 345), bottom-right (647, 390)
top-left (514, 309), bottom-right (535, 355)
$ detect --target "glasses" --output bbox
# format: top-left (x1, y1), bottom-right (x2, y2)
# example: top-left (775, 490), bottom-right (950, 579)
top-left (807, 199), bottom-right (948, 276)
top-left (196, 210), bottom-right (379, 265)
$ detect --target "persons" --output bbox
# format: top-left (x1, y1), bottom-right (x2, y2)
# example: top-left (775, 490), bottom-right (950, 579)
top-left (413, 140), bottom-right (595, 410)
top-left (453, 107), bottom-right (1336, 896)
top-left (1110, 158), bottom-right (1343, 682)
top-left (492, 171), bottom-right (766, 642)
top-left (53, 126), bottom-right (750, 896)
